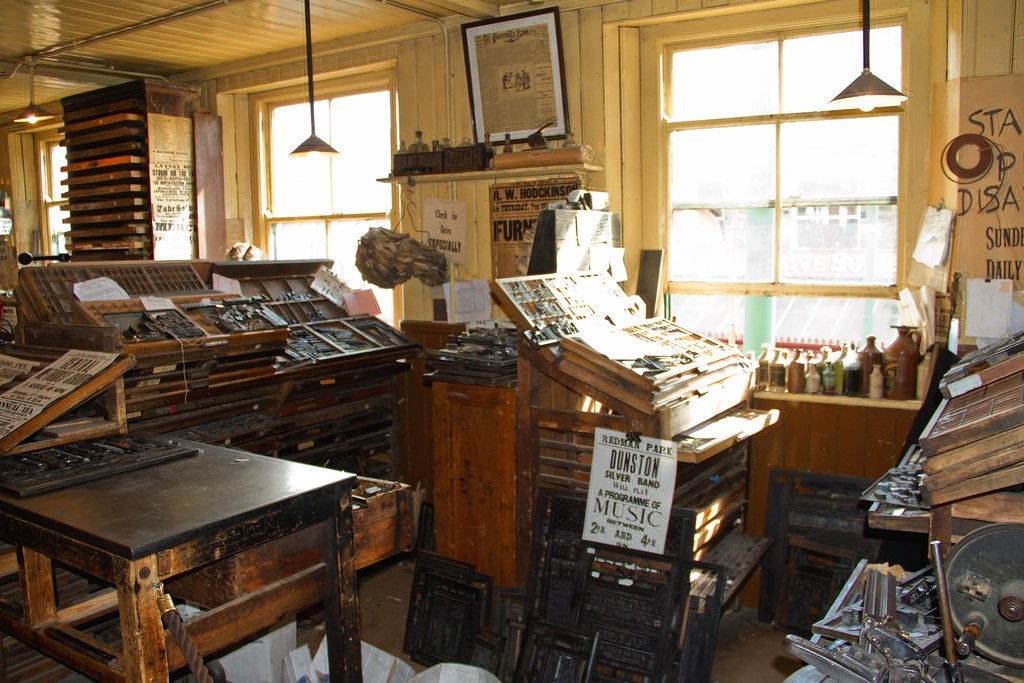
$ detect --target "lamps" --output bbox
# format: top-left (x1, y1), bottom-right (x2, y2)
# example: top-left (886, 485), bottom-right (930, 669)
top-left (286, 0), bottom-right (340, 159)
top-left (13, 55), bottom-right (56, 123)
top-left (826, 0), bottom-right (910, 113)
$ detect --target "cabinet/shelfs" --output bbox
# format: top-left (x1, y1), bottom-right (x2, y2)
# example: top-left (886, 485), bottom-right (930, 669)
top-left (429, 378), bottom-right (517, 595)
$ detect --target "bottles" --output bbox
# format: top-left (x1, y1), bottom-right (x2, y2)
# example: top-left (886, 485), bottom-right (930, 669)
top-left (563, 132), bottom-right (577, 148)
top-left (396, 140), bottom-right (408, 154)
top-left (432, 137), bottom-right (453, 153)
top-left (484, 133), bottom-right (495, 169)
top-left (503, 134), bottom-right (513, 153)
top-left (715, 322), bottom-right (933, 400)
top-left (407, 131), bottom-right (430, 153)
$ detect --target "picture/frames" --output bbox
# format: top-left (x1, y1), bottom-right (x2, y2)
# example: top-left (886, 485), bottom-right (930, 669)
top-left (460, 5), bottom-right (571, 147)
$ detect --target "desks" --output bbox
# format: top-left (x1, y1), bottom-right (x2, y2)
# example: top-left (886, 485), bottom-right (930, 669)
top-left (0, 431), bottom-right (364, 683)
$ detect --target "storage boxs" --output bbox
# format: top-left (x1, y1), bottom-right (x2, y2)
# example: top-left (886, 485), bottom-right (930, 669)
top-left (165, 479), bottom-right (417, 609)
